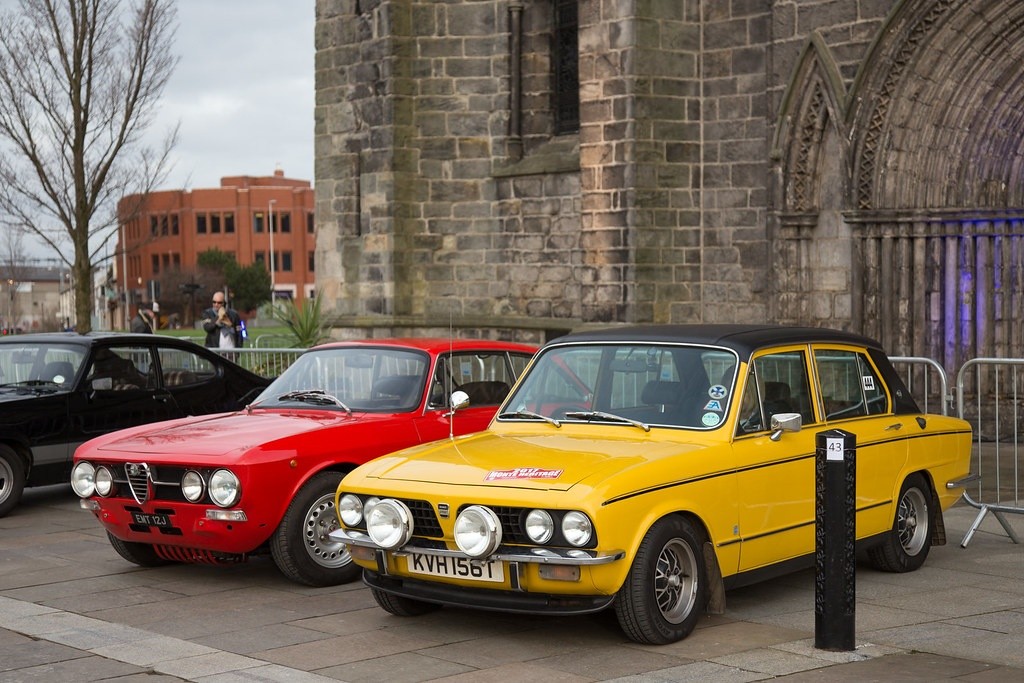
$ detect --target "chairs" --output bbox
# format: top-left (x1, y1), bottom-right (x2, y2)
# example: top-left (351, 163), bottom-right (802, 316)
top-left (39, 361), bottom-right (75, 383)
top-left (636, 381), bottom-right (684, 426)
top-left (106, 359), bottom-right (137, 384)
top-left (754, 383), bottom-right (794, 425)
top-left (371, 375), bottom-right (418, 403)
top-left (163, 369), bottom-right (197, 386)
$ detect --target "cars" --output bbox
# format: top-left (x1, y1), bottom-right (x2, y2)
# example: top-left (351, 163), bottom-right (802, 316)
top-left (0, 330), bottom-right (281, 521)
top-left (68, 338), bottom-right (595, 588)
top-left (327, 321), bottom-right (982, 648)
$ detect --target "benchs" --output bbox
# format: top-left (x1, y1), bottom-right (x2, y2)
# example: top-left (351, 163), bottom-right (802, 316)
top-left (452, 381), bottom-right (509, 405)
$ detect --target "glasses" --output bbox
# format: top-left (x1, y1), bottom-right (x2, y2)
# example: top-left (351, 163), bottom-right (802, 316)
top-left (212, 300), bottom-right (225, 305)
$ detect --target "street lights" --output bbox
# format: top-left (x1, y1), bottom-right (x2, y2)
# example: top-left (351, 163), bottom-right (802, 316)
top-left (267, 198), bottom-right (276, 320)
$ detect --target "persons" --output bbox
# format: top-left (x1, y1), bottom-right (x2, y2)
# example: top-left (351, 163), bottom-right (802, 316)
top-left (708, 366), bottom-right (776, 433)
top-left (130, 302), bottom-right (160, 334)
top-left (201, 292), bottom-right (244, 364)
